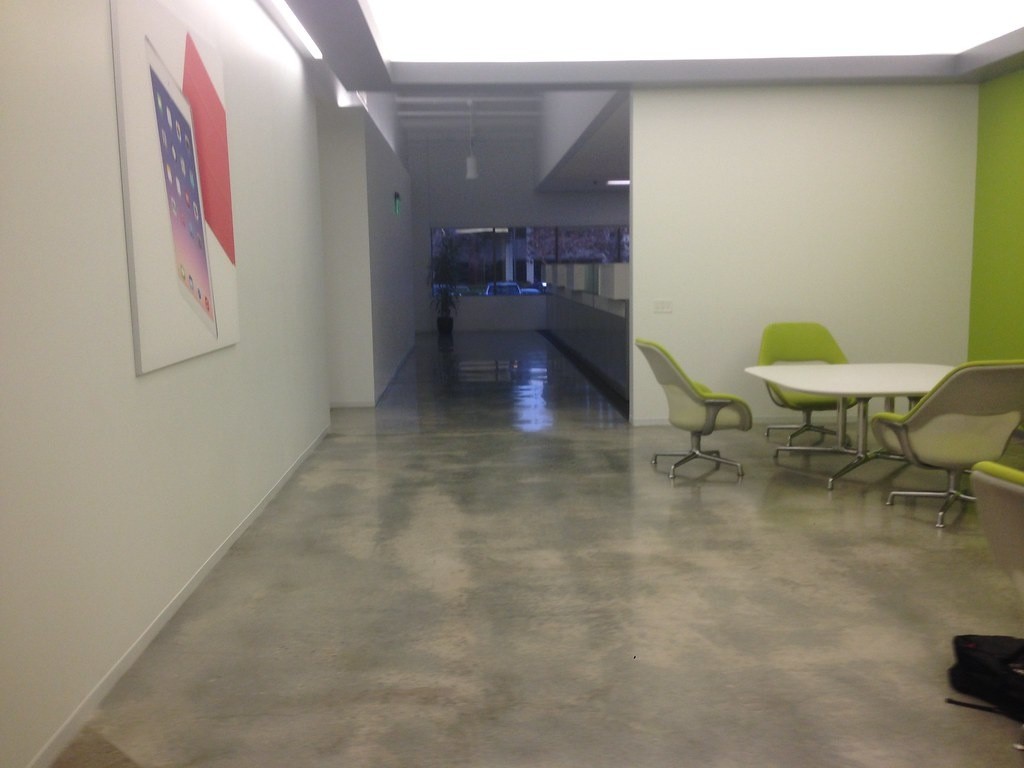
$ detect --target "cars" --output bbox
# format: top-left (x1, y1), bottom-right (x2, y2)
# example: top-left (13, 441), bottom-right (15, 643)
top-left (513, 288), bottom-right (540, 294)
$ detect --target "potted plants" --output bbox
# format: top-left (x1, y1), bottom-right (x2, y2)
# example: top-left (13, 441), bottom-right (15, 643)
top-left (426, 238), bottom-right (470, 332)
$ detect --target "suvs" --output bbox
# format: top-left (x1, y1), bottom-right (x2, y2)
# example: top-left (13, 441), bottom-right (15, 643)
top-left (483, 281), bottom-right (522, 296)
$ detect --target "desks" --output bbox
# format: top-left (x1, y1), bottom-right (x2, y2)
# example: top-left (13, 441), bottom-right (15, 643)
top-left (744, 360), bottom-right (956, 492)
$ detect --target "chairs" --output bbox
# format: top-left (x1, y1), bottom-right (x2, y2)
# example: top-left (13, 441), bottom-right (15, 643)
top-left (971, 458), bottom-right (1024, 753)
top-left (632, 340), bottom-right (753, 481)
top-left (871, 360), bottom-right (1024, 529)
top-left (759, 321), bottom-right (858, 449)
